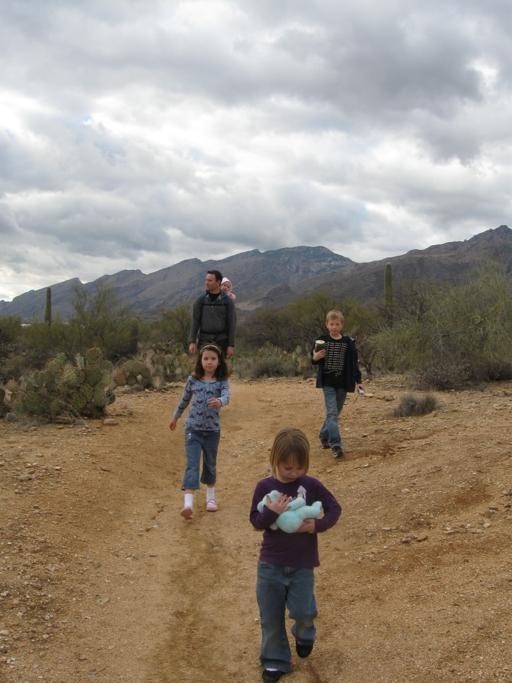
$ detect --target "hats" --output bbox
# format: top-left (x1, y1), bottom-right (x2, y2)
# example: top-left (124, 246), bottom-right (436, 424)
top-left (221, 276), bottom-right (231, 284)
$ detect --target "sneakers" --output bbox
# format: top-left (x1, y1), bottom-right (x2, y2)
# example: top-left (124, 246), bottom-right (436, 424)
top-left (205, 497), bottom-right (219, 513)
top-left (319, 435), bottom-right (330, 450)
top-left (180, 505), bottom-right (194, 521)
top-left (261, 667), bottom-right (284, 682)
top-left (331, 447), bottom-right (343, 459)
top-left (294, 640), bottom-right (313, 658)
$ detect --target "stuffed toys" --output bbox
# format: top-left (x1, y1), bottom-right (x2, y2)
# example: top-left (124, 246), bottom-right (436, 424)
top-left (256, 484), bottom-right (323, 534)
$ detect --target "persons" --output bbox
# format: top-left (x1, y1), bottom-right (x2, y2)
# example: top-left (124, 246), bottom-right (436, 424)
top-left (248, 424), bottom-right (342, 682)
top-left (220, 274), bottom-right (237, 301)
top-left (169, 342), bottom-right (232, 521)
top-left (310, 307), bottom-right (365, 458)
top-left (188, 268), bottom-right (237, 384)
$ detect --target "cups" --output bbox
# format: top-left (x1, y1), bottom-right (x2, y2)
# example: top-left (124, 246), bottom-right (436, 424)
top-left (314, 340), bottom-right (325, 354)
